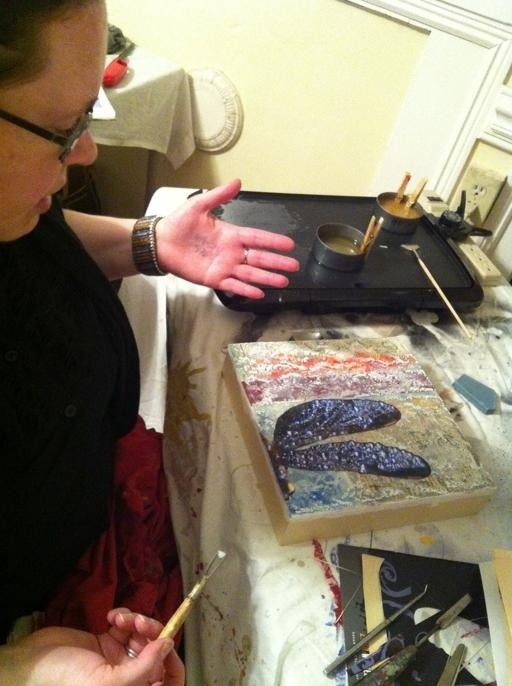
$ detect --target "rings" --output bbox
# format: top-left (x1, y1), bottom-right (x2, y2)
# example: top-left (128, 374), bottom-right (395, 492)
top-left (244, 248), bottom-right (248, 265)
top-left (124, 647), bottom-right (138, 658)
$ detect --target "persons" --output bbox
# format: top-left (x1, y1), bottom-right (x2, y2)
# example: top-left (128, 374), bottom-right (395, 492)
top-left (0, 1), bottom-right (298, 686)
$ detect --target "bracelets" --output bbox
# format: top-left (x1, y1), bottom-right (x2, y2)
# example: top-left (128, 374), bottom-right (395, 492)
top-left (131, 215), bottom-right (167, 277)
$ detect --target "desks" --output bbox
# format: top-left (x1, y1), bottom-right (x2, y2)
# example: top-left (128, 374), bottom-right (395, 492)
top-left (152, 183), bottom-right (510, 686)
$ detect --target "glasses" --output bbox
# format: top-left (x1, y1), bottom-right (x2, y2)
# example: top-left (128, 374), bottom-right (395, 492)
top-left (0, 108), bottom-right (92, 160)
